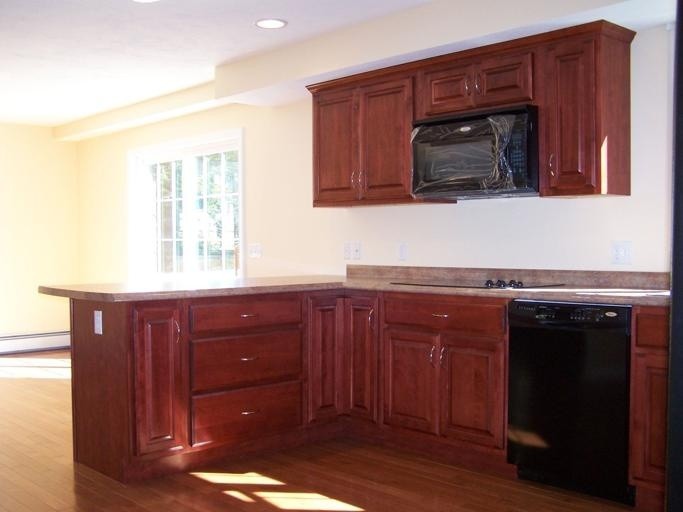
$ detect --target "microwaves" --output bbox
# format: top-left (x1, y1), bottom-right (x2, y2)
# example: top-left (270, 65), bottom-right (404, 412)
top-left (407, 108), bottom-right (540, 203)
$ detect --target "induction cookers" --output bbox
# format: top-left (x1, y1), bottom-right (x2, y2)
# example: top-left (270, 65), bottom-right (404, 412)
top-left (390, 279), bottom-right (566, 289)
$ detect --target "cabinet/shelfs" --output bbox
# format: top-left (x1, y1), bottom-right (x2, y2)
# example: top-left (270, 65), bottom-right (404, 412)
top-left (384, 297), bottom-right (505, 450)
top-left (312, 78), bottom-right (456, 205)
top-left (412, 50), bottom-right (534, 114)
top-left (307, 291), bottom-right (381, 424)
top-left (628, 303), bottom-right (670, 495)
top-left (187, 296), bottom-right (304, 451)
top-left (71, 297), bottom-right (184, 484)
top-left (539, 35), bottom-right (631, 195)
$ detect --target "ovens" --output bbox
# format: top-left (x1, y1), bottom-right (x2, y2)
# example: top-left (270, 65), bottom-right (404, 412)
top-left (506, 295), bottom-right (636, 507)
